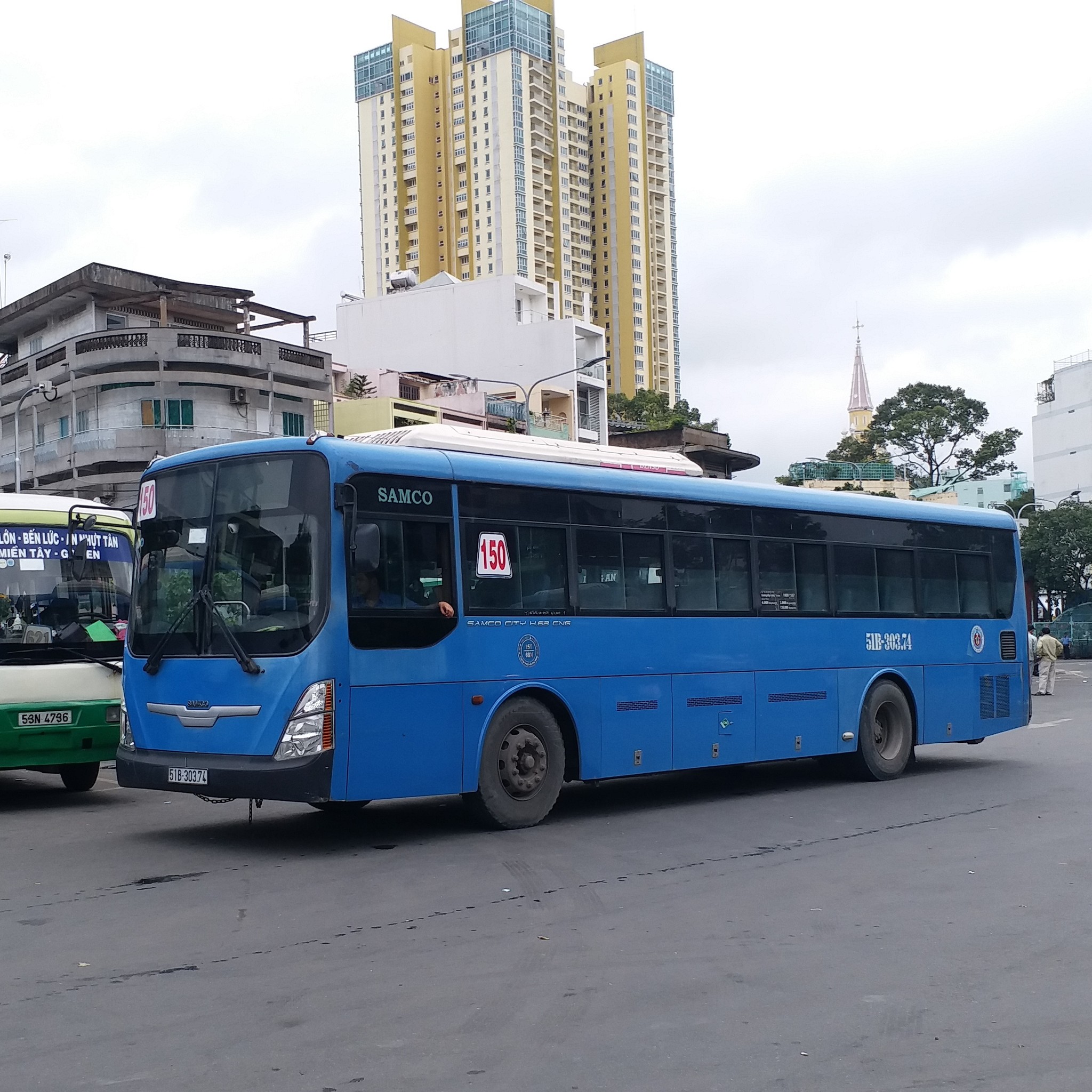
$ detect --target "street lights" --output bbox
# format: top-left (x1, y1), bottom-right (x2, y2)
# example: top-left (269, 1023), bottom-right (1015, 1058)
top-left (1034, 490), bottom-right (1081, 614)
top-left (12, 378), bottom-right (58, 494)
top-left (445, 354), bottom-right (610, 436)
top-left (803, 449), bottom-right (918, 491)
top-left (985, 500), bottom-right (1045, 526)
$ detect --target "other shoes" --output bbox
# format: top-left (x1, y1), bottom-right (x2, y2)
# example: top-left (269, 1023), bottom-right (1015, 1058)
top-left (1032, 691), bottom-right (1052, 696)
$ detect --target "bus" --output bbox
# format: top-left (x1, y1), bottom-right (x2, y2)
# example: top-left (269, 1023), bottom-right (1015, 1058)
top-left (0, 491), bottom-right (137, 790)
top-left (65, 420), bottom-right (1033, 826)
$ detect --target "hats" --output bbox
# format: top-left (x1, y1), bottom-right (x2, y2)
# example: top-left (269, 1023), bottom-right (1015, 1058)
top-left (1028, 623), bottom-right (1036, 631)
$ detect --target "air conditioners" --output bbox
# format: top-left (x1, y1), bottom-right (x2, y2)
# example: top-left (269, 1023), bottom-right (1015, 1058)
top-left (230, 387), bottom-right (250, 404)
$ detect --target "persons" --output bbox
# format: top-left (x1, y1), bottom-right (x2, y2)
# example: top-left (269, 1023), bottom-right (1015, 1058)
top-left (140, 531), bottom-right (205, 611)
top-left (1085, 629), bottom-right (1090, 639)
top-left (349, 566), bottom-right (454, 619)
top-left (1034, 628), bottom-right (1064, 696)
top-left (1061, 633), bottom-right (1071, 660)
top-left (1027, 624), bottom-right (1037, 688)
top-left (29, 591), bottom-right (79, 631)
